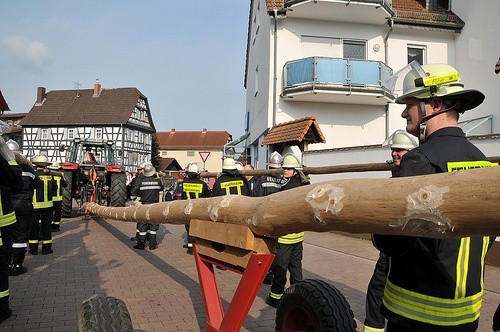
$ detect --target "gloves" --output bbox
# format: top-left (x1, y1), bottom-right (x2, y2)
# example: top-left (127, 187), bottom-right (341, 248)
top-left (132, 199), bottom-right (142, 206)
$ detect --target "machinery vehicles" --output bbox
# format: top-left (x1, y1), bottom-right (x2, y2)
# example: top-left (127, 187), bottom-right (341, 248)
top-left (59, 136), bottom-right (128, 218)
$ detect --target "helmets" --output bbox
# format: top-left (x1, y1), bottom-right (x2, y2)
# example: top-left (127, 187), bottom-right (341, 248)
top-left (186, 162), bottom-right (200, 173)
top-left (222, 158), bottom-right (237, 169)
top-left (234, 161), bottom-right (244, 170)
top-left (269, 151), bottom-right (283, 168)
top-left (396, 63), bottom-right (485, 113)
top-left (0, 119), bottom-right (12, 136)
top-left (6, 139), bottom-right (22, 153)
top-left (32, 153), bottom-right (50, 166)
top-left (281, 153), bottom-right (303, 170)
top-left (142, 164), bottom-right (156, 177)
top-left (48, 161), bottom-right (61, 169)
top-left (391, 131), bottom-right (420, 149)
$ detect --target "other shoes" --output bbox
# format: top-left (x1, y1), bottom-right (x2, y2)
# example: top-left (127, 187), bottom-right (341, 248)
top-left (186, 248), bottom-right (194, 255)
top-left (50, 226), bottom-right (60, 232)
top-left (7, 263), bottom-right (27, 276)
top-left (149, 245), bottom-right (156, 250)
top-left (215, 265), bottom-right (227, 271)
top-left (28, 248), bottom-right (54, 255)
top-left (301, 165), bottom-right (311, 183)
top-left (0, 308), bottom-right (12, 324)
top-left (133, 245), bottom-right (144, 250)
top-left (262, 276), bottom-right (272, 285)
top-left (265, 292), bottom-right (280, 308)
top-left (183, 243), bottom-right (193, 248)
top-left (130, 236), bottom-right (138, 241)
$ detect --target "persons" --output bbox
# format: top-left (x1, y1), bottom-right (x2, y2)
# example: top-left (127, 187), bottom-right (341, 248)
top-left (126, 164), bottom-right (164, 249)
top-left (213, 151), bottom-right (311, 308)
top-left (165, 163), bottom-right (211, 255)
top-left (0, 120), bottom-right (36, 321)
top-left (29, 154), bottom-right (67, 255)
top-left (363, 62), bottom-right (500, 332)
